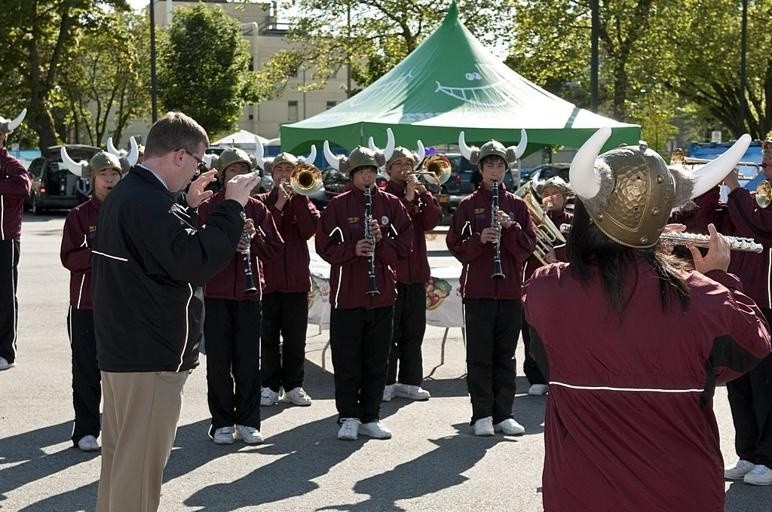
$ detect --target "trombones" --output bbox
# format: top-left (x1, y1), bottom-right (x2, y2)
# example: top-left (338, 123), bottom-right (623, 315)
top-left (279, 157), bottom-right (325, 202)
top-left (400, 152), bottom-right (453, 195)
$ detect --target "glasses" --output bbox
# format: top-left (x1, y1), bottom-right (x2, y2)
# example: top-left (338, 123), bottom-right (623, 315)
top-left (174, 146), bottom-right (206, 168)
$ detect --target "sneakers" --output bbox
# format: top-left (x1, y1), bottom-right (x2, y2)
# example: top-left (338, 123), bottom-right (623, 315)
top-left (283, 387), bottom-right (312, 406)
top-left (338, 418), bottom-right (361, 440)
top-left (0, 356), bottom-right (16, 370)
top-left (383, 384), bottom-right (431, 401)
top-left (743, 464), bottom-right (772, 485)
top-left (260, 386), bottom-right (279, 406)
top-left (78, 435), bottom-right (99, 451)
top-left (474, 416), bottom-right (495, 436)
top-left (724, 459), bottom-right (757, 478)
top-left (359, 422), bottom-right (392, 439)
top-left (528, 384), bottom-right (549, 396)
top-left (494, 418), bottom-right (525, 435)
top-left (214, 425), bottom-right (263, 444)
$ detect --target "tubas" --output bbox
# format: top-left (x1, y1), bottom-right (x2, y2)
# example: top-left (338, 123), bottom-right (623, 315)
top-left (513, 181), bottom-right (566, 265)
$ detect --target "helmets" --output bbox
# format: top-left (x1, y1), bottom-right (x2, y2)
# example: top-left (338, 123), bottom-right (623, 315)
top-left (458, 129), bottom-right (528, 167)
top-left (531, 172), bottom-right (575, 202)
top-left (323, 128), bottom-right (426, 177)
top-left (206, 137), bottom-right (265, 184)
top-left (568, 127), bottom-right (752, 249)
top-left (265, 144), bottom-right (318, 177)
top-left (60, 136), bottom-right (144, 188)
top-left (1, 108), bottom-right (28, 134)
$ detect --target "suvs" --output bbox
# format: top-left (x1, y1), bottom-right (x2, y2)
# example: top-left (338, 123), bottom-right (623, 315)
top-left (436, 152), bottom-right (513, 200)
top-left (514, 163), bottom-right (572, 191)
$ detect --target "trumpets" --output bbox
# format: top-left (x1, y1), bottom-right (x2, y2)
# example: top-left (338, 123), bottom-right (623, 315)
top-left (671, 148), bottom-right (767, 180)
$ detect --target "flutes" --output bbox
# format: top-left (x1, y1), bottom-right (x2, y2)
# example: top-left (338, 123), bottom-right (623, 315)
top-left (560, 222), bottom-right (762, 256)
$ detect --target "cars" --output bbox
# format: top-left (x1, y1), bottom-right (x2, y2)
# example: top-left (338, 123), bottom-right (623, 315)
top-left (247, 160), bottom-right (273, 194)
top-left (25, 142), bottom-right (107, 213)
top-left (257, 156), bottom-right (275, 194)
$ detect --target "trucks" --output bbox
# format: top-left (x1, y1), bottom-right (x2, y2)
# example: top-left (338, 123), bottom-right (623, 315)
top-left (205, 145), bottom-right (226, 160)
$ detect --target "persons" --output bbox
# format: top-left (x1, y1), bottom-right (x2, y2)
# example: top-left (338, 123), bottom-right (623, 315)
top-left (314, 146), bottom-right (413, 440)
top-left (683, 138), bottom-right (771, 487)
top-left (60, 151), bottom-right (123, 449)
top-left (522, 139), bottom-right (772, 511)
top-left (191, 148), bottom-right (286, 443)
top-left (1, 110), bottom-right (38, 379)
top-left (447, 140), bottom-right (536, 434)
top-left (382, 150), bottom-right (443, 401)
top-left (253, 150), bottom-right (321, 405)
top-left (90, 112), bottom-right (260, 510)
